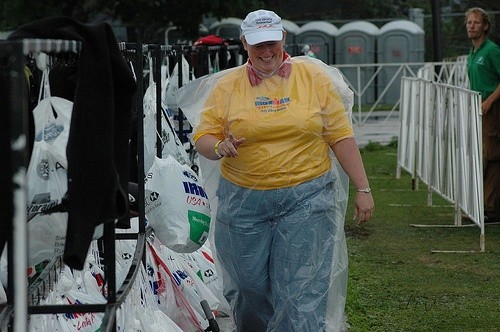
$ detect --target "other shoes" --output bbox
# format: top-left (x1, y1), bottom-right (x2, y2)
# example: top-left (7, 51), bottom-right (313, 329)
top-left (463, 213), bottom-right (469, 219)
top-left (484, 215), bottom-right (500, 222)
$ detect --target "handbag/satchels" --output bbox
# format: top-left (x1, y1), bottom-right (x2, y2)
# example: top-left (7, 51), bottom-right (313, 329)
top-left (0, 49), bottom-right (230, 332)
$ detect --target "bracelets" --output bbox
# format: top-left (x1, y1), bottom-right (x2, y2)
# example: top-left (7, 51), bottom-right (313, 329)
top-left (356, 184), bottom-right (372, 193)
top-left (214, 139), bottom-right (223, 158)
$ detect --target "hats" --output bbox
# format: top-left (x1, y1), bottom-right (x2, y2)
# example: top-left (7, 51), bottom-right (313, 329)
top-left (301, 45), bottom-right (310, 52)
top-left (240, 10), bottom-right (287, 46)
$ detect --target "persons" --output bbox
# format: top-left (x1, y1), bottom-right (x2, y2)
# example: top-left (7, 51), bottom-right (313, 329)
top-left (193, 9), bottom-right (376, 332)
top-left (461, 7), bottom-right (500, 223)
